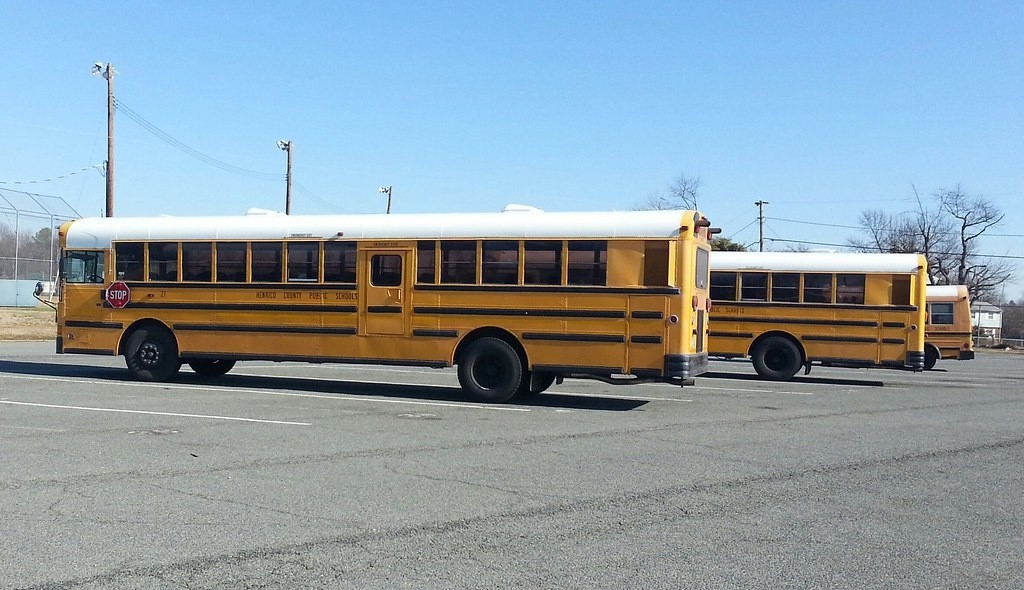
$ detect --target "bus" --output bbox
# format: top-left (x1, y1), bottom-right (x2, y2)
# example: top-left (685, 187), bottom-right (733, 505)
top-left (924, 285), bottom-right (975, 370)
top-left (707, 252), bottom-right (928, 381)
top-left (32, 207), bottom-right (723, 405)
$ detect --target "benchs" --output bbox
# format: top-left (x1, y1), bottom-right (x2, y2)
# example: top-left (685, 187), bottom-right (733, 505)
top-left (167, 269), bottom-right (603, 284)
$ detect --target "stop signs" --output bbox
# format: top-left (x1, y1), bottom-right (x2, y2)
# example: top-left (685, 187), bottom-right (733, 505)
top-left (105, 281), bottom-right (130, 308)
